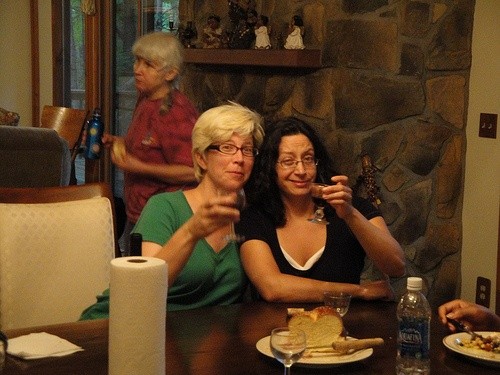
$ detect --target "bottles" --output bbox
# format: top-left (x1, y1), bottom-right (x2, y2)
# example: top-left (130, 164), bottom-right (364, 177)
top-left (395, 277), bottom-right (432, 375)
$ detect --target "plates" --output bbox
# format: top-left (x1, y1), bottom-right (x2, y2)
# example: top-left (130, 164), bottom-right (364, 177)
top-left (442, 331), bottom-right (500, 363)
top-left (256, 333), bottom-right (374, 364)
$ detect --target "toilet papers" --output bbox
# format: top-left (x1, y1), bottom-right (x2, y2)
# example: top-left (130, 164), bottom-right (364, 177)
top-left (108, 256), bottom-right (169, 375)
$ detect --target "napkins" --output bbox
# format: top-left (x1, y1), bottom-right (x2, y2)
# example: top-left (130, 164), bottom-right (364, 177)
top-left (6, 332), bottom-right (85, 360)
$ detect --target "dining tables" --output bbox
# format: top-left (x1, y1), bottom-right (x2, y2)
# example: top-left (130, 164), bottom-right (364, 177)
top-left (0, 301), bottom-right (500, 375)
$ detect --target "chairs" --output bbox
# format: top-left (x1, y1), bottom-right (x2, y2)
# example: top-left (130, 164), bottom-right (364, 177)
top-left (0, 179), bottom-right (125, 331)
top-left (42, 105), bottom-right (90, 185)
top-left (0, 123), bottom-right (72, 189)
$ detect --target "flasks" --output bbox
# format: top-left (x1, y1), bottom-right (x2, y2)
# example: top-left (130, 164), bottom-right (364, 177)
top-left (83, 107), bottom-right (105, 160)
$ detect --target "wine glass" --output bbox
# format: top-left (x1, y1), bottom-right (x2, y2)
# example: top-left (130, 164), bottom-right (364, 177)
top-left (217, 186), bottom-right (246, 242)
top-left (269, 327), bottom-right (306, 375)
top-left (307, 183), bottom-right (331, 225)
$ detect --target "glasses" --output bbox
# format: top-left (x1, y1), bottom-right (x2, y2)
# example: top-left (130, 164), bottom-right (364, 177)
top-left (275, 156), bottom-right (320, 169)
top-left (205, 143), bottom-right (259, 157)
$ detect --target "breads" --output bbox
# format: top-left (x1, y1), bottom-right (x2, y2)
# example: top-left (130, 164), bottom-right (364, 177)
top-left (288, 306), bottom-right (344, 352)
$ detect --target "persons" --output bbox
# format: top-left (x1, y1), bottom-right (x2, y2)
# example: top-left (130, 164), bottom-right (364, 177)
top-left (96, 32), bottom-right (205, 276)
top-left (74, 100), bottom-right (266, 319)
top-left (437, 301), bottom-right (500, 336)
top-left (240, 113), bottom-right (409, 306)
top-left (202, 10), bottom-right (306, 50)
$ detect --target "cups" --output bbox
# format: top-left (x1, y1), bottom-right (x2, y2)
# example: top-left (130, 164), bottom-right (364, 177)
top-left (324, 293), bottom-right (351, 317)
